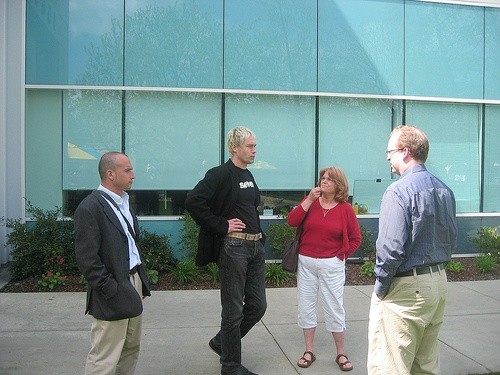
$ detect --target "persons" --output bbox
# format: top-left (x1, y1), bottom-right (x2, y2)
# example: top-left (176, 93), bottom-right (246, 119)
top-left (286, 166), bottom-right (363, 372)
top-left (368, 124), bottom-right (458, 375)
top-left (183, 125), bottom-right (269, 375)
top-left (73, 151), bottom-right (151, 375)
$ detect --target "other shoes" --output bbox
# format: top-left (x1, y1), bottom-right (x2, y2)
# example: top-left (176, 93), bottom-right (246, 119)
top-left (221, 365), bottom-right (257, 375)
top-left (209, 339), bottom-right (221, 356)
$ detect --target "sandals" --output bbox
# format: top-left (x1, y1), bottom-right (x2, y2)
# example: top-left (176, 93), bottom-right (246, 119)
top-left (297, 351), bottom-right (315, 368)
top-left (336, 354), bottom-right (353, 371)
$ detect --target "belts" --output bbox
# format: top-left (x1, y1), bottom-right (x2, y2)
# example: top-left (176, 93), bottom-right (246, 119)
top-left (130, 266), bottom-right (138, 275)
top-left (395, 263), bottom-right (445, 277)
top-left (226, 231), bottom-right (262, 241)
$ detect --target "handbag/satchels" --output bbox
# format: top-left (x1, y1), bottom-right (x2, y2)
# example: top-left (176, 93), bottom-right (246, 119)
top-left (282, 223), bottom-right (303, 273)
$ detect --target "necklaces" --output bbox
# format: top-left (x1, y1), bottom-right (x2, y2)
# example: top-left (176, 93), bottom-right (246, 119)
top-left (320, 201), bottom-right (332, 216)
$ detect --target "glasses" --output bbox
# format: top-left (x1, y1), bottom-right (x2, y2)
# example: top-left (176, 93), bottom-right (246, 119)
top-left (385, 148), bottom-right (403, 154)
